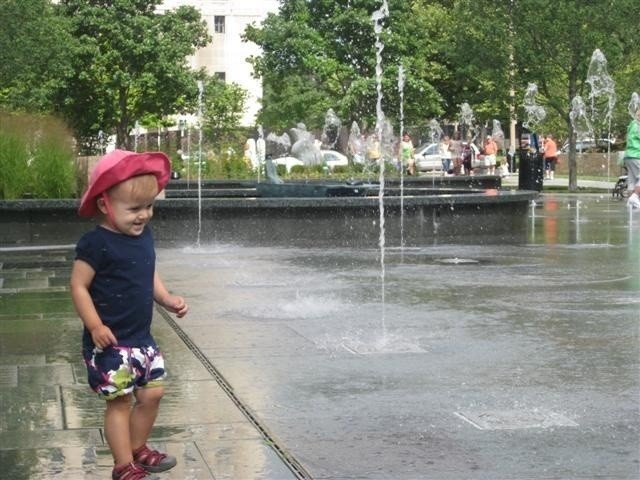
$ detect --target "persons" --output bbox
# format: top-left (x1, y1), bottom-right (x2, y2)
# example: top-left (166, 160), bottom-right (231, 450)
top-left (541, 194), bottom-right (561, 256)
top-left (365, 131), bottom-right (383, 174)
top-left (543, 134), bottom-right (558, 181)
top-left (461, 144), bottom-right (472, 176)
top-left (397, 131), bottom-right (416, 178)
top-left (497, 157), bottom-right (509, 178)
top-left (447, 133), bottom-right (466, 176)
top-left (351, 134), bottom-right (367, 167)
top-left (438, 133), bottom-right (453, 179)
top-left (623, 108), bottom-right (640, 210)
top-left (68, 148), bottom-right (187, 480)
top-left (477, 134), bottom-right (498, 176)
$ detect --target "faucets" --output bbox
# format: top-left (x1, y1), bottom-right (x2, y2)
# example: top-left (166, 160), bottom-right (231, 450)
top-left (112, 445), bottom-right (178, 480)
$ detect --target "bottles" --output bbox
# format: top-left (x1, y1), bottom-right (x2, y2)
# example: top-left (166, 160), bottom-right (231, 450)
top-left (77, 147), bottom-right (171, 215)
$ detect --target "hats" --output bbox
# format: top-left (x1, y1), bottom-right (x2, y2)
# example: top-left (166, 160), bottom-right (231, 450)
top-left (404, 122), bottom-right (481, 175)
top-left (557, 138), bottom-right (611, 155)
top-left (273, 151), bottom-right (349, 172)
